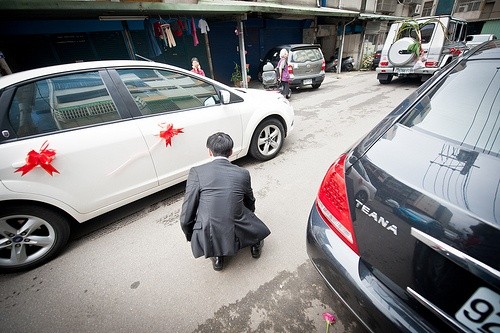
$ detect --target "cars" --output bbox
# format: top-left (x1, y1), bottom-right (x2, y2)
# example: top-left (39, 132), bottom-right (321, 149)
top-left (0, 59), bottom-right (296, 273)
top-left (305, 39), bottom-right (500, 333)
top-left (465, 33), bottom-right (499, 49)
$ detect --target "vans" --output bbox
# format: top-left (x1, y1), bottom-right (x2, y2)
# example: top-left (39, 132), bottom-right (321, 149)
top-left (257, 43), bottom-right (326, 92)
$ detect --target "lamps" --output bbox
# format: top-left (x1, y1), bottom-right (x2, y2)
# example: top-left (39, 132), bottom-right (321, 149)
top-left (99, 16), bottom-right (148, 21)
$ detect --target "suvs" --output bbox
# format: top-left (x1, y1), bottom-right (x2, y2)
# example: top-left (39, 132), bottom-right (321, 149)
top-left (376, 14), bottom-right (470, 87)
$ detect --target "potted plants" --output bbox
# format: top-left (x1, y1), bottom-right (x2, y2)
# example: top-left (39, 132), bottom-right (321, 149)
top-left (231, 64), bottom-right (242, 87)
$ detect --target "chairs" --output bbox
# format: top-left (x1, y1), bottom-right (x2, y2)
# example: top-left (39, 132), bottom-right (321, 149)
top-left (262, 70), bottom-right (282, 92)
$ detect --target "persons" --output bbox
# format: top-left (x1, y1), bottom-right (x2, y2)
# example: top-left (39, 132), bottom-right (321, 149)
top-left (180, 133), bottom-right (271, 270)
top-left (190, 58), bottom-right (205, 76)
top-left (275, 48), bottom-right (292, 98)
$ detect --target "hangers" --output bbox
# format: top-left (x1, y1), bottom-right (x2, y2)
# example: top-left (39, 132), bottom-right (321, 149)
top-left (158, 15), bottom-right (193, 24)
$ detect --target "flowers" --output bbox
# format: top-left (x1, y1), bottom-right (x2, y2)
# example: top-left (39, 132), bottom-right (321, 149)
top-left (363, 53), bottom-right (375, 69)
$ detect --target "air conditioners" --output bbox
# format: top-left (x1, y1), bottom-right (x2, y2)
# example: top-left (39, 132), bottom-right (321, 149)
top-left (457, 5), bottom-right (469, 12)
